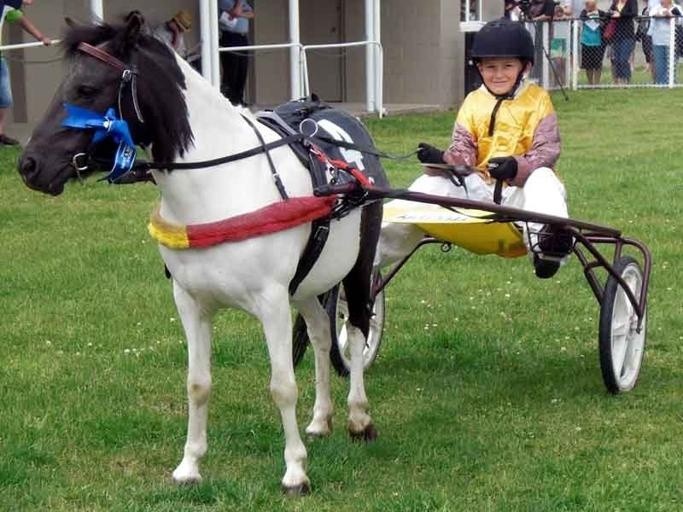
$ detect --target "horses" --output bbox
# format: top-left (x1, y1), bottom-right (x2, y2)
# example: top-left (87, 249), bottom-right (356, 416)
top-left (16, 11), bottom-right (384, 502)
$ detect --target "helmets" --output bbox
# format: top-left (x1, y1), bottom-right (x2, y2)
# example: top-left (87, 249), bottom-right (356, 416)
top-left (469, 17), bottom-right (535, 59)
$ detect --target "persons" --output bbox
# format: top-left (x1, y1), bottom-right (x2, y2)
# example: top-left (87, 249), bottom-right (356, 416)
top-left (365, 17), bottom-right (574, 280)
top-left (0, 0), bottom-right (51, 145)
top-left (504, 2), bottom-right (682, 89)
top-left (216, 0), bottom-right (256, 107)
top-left (150, 9), bottom-right (194, 62)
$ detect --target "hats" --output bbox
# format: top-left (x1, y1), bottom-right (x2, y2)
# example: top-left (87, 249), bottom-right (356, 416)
top-left (173, 8), bottom-right (193, 33)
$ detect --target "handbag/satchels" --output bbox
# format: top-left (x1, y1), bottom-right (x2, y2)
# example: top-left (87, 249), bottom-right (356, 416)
top-left (603, 17), bottom-right (616, 41)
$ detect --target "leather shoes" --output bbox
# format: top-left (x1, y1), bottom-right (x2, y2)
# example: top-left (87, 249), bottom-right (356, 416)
top-left (0, 134), bottom-right (20, 145)
top-left (532, 221), bottom-right (573, 279)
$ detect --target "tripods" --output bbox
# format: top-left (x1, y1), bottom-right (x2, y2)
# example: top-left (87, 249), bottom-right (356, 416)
top-left (517, 13), bottom-right (569, 102)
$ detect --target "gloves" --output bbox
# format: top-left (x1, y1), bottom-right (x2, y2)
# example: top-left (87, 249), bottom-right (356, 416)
top-left (416, 142), bottom-right (446, 169)
top-left (487, 156), bottom-right (517, 205)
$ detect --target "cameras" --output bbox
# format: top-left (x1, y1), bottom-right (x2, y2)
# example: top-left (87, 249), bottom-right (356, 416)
top-left (519, 1), bottom-right (530, 14)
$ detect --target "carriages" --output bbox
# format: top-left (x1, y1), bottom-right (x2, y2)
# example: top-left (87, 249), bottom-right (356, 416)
top-left (16, 8), bottom-right (652, 497)
